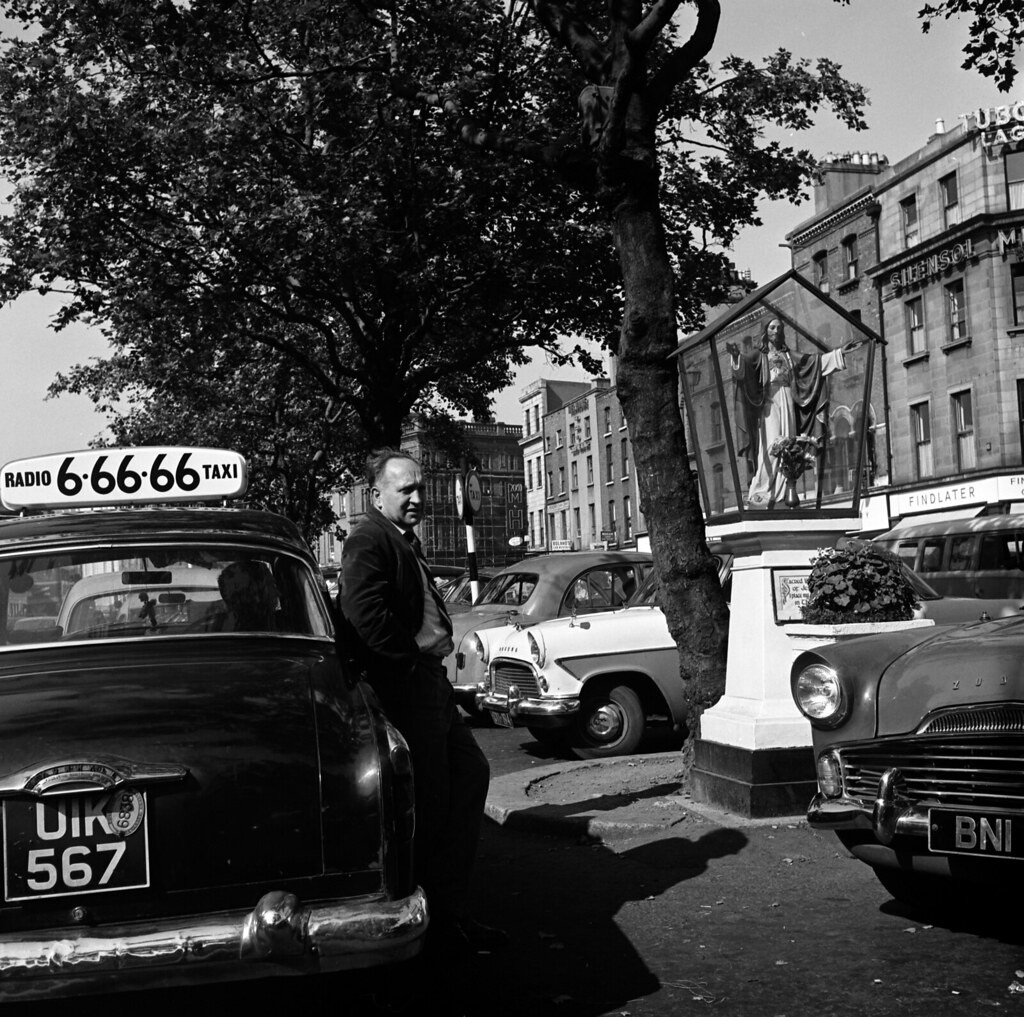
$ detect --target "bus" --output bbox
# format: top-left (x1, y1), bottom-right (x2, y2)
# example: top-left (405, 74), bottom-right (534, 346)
top-left (872, 512), bottom-right (1024, 599)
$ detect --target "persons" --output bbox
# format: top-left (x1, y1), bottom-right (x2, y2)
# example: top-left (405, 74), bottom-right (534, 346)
top-left (725, 316), bottom-right (863, 504)
top-left (336, 449), bottom-right (511, 948)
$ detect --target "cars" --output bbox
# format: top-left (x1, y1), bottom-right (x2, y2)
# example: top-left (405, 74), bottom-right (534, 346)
top-left (467, 537), bottom-right (1024, 760)
top-left (787, 607), bottom-right (1024, 911)
top-left (54, 563), bottom-right (224, 636)
top-left (320, 549), bottom-right (652, 721)
top-left (0, 446), bottom-right (431, 1006)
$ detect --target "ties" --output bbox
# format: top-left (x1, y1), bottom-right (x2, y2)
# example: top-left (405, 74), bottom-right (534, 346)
top-left (404, 531), bottom-right (454, 637)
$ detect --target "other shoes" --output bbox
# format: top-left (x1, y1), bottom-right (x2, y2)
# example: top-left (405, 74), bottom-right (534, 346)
top-left (415, 919), bottom-right (511, 961)
